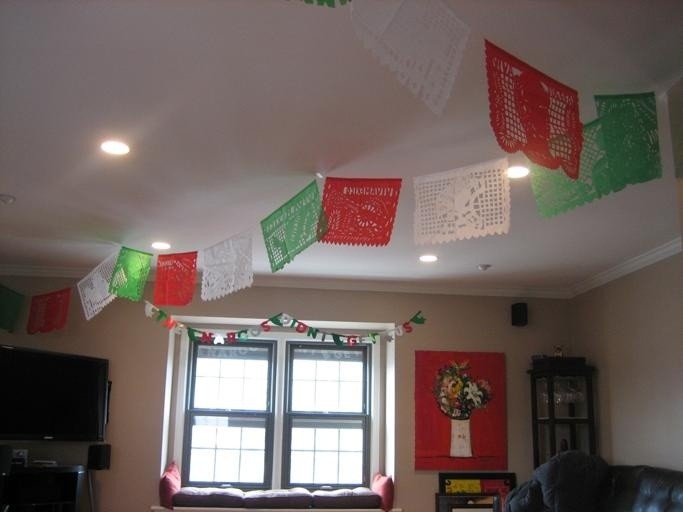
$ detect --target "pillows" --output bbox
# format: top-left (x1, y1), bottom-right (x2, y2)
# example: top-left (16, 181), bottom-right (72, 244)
top-left (535, 443), bottom-right (609, 511)
top-left (161, 453), bottom-right (395, 511)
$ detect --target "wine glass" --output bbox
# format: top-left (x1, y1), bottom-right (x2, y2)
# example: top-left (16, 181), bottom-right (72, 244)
top-left (540, 375), bottom-right (586, 406)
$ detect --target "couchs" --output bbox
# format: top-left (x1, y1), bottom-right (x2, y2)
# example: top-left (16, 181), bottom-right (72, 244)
top-left (505, 456), bottom-right (683, 511)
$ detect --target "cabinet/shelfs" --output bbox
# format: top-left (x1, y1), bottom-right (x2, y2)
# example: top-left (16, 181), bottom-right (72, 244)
top-left (2, 458), bottom-right (85, 512)
top-left (529, 367), bottom-right (598, 470)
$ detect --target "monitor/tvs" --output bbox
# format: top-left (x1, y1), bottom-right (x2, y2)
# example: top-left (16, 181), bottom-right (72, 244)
top-left (0, 344), bottom-right (109, 441)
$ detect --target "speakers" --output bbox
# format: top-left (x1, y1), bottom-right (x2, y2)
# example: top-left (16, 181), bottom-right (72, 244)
top-left (511, 302), bottom-right (528, 327)
top-left (86, 444), bottom-right (111, 471)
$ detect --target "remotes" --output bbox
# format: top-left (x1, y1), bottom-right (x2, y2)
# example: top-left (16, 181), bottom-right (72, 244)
top-left (32, 459), bottom-right (55, 464)
